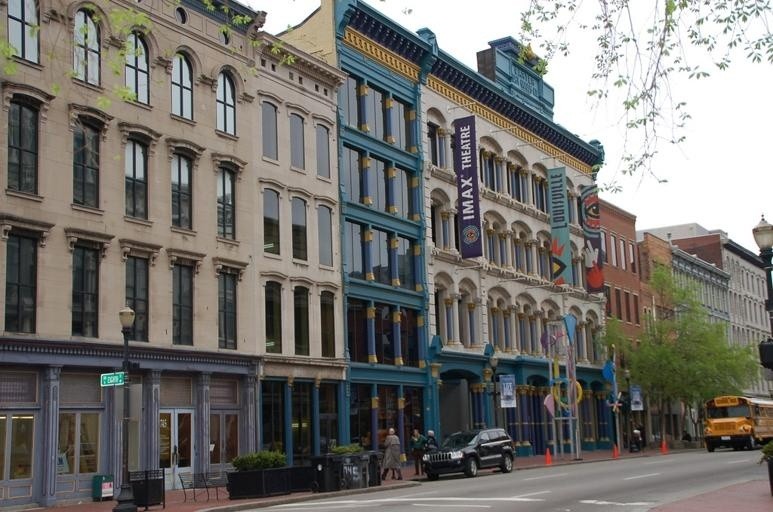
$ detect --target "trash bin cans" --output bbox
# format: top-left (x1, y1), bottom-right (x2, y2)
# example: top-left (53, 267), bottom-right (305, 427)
top-left (128, 469), bottom-right (165, 511)
top-left (309, 451), bottom-right (384, 493)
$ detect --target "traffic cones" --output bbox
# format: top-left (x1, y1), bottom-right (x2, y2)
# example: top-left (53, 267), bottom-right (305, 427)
top-left (659, 438), bottom-right (669, 456)
top-left (612, 444), bottom-right (618, 459)
top-left (543, 448), bottom-right (552, 465)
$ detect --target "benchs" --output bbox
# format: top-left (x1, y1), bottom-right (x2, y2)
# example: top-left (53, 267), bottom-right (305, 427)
top-left (177, 470), bottom-right (229, 504)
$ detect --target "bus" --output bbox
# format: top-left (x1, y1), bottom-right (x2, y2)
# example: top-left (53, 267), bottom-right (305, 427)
top-left (700, 393), bottom-right (772, 455)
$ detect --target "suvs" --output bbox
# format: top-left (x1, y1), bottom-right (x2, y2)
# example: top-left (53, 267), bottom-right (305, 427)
top-left (420, 427), bottom-right (518, 481)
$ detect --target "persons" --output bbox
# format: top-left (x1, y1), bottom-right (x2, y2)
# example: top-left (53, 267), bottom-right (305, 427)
top-left (680, 429), bottom-right (692, 441)
top-left (379, 427), bottom-right (402, 480)
top-left (631, 418), bottom-right (646, 451)
top-left (423, 429), bottom-right (439, 452)
top-left (407, 429), bottom-right (428, 475)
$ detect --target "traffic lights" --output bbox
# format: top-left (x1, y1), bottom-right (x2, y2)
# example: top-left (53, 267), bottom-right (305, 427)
top-left (756, 339), bottom-right (772, 370)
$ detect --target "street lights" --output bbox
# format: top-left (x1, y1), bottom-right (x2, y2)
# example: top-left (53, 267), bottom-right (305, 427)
top-left (488, 350), bottom-right (500, 428)
top-left (112, 297), bottom-right (140, 512)
top-left (749, 211), bottom-right (772, 345)
top-left (622, 367), bottom-right (633, 453)
